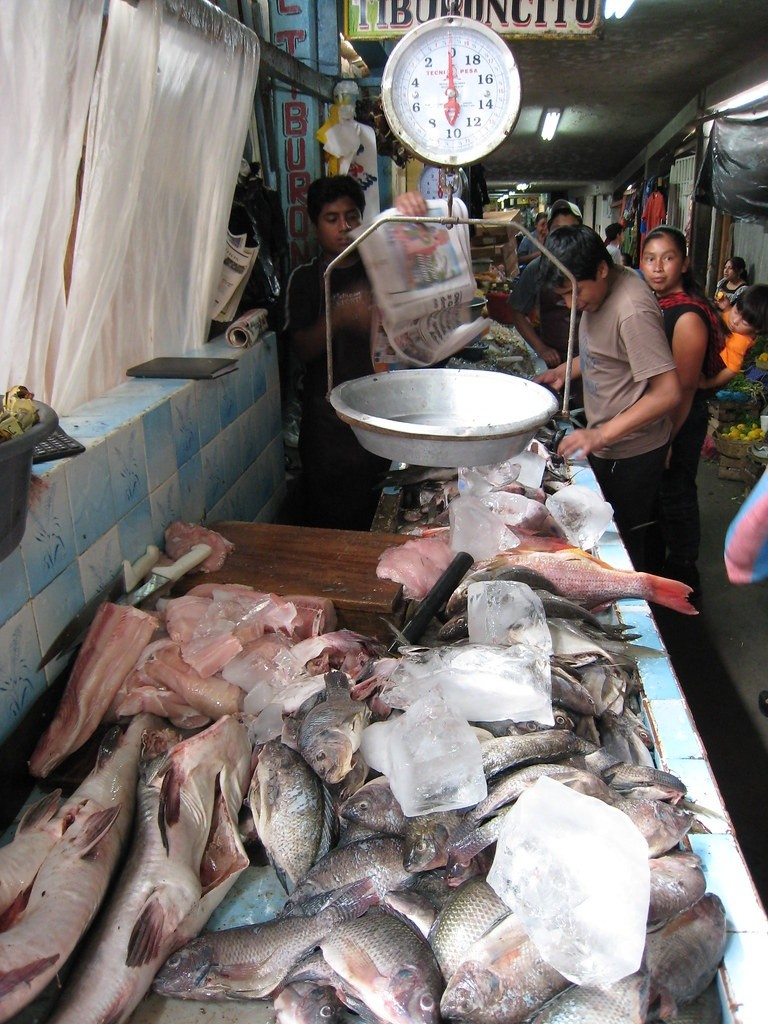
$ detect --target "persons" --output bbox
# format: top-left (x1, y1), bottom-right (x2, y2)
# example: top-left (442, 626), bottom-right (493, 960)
top-left (280, 175), bottom-right (427, 528)
top-left (508, 198), bottom-right (767, 720)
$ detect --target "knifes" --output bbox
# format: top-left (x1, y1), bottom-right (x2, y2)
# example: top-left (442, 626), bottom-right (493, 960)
top-left (55, 542), bottom-right (213, 662)
top-left (36, 544), bottom-right (160, 674)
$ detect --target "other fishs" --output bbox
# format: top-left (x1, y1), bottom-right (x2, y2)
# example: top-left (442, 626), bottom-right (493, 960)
top-left (0, 406), bottom-right (726, 1024)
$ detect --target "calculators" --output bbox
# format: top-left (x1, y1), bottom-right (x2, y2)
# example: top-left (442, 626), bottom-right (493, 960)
top-left (31, 425), bottom-right (85, 465)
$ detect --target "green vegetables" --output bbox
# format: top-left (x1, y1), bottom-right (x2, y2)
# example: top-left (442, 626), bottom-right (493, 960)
top-left (725, 372), bottom-right (764, 397)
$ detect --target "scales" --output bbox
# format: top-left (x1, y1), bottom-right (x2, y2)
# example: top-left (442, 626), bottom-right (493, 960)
top-left (320, 0), bottom-right (577, 469)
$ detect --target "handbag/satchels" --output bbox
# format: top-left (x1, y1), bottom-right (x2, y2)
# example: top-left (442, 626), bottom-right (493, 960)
top-left (724, 461), bottom-right (768, 585)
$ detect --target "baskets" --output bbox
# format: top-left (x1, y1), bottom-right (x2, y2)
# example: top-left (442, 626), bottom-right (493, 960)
top-left (712, 430), bottom-right (766, 458)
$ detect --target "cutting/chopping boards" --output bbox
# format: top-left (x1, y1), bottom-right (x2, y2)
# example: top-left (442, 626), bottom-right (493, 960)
top-left (174, 519), bottom-right (424, 614)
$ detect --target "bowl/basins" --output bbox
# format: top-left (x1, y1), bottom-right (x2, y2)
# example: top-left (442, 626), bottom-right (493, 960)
top-left (0, 394), bottom-right (60, 565)
top-left (484, 293), bottom-right (512, 324)
top-left (468, 296), bottom-right (488, 321)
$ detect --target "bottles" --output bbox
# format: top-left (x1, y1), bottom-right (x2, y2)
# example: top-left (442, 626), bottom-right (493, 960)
top-left (489, 274), bottom-right (519, 293)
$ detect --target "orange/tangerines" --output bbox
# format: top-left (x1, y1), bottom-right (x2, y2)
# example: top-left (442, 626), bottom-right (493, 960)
top-left (721, 422), bottom-right (766, 441)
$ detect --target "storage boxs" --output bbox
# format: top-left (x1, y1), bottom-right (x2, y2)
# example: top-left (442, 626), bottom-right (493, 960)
top-left (471, 210), bottom-right (521, 276)
top-left (707, 401), bottom-right (768, 491)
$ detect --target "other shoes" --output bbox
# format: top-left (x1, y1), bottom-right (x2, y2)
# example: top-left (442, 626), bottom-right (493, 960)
top-left (759, 690), bottom-right (768, 716)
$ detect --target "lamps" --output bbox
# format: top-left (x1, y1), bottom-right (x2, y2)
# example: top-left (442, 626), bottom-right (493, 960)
top-left (541, 107), bottom-right (562, 141)
top-left (604, 0), bottom-right (635, 19)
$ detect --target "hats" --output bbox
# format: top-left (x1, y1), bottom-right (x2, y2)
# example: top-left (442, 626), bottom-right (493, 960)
top-left (547, 199), bottom-right (582, 224)
